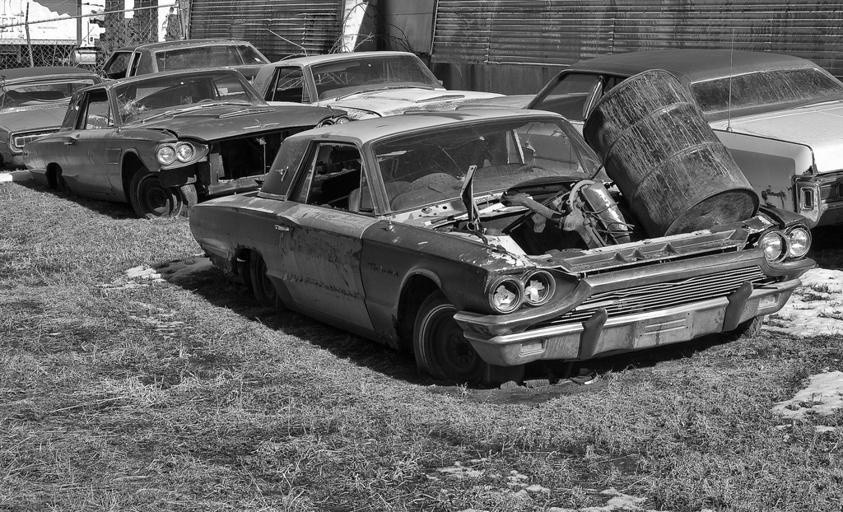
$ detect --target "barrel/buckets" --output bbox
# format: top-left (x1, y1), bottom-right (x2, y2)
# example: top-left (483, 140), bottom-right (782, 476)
top-left (582, 67), bottom-right (761, 235)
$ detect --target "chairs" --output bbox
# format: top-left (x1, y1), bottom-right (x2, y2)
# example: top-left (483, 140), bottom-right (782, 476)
top-left (347, 181), bottom-right (411, 212)
top-left (449, 140), bottom-right (506, 175)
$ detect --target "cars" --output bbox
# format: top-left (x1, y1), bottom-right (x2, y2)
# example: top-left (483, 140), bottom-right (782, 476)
top-left (456, 46), bottom-right (843, 239)
top-left (255, 50), bottom-right (507, 121)
top-left (186, 105), bottom-right (817, 388)
top-left (22, 67), bottom-right (349, 223)
top-left (0, 66), bottom-right (149, 168)
top-left (101, 40), bottom-right (271, 81)
